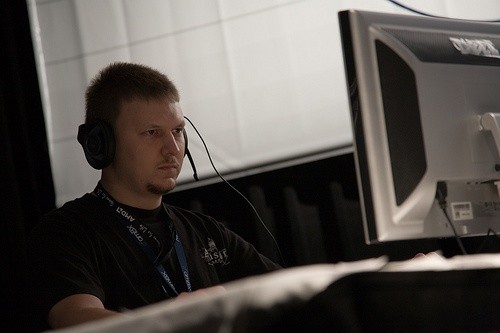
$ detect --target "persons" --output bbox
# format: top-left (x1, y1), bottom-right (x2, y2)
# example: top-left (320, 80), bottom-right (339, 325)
top-left (44, 64), bottom-right (300, 329)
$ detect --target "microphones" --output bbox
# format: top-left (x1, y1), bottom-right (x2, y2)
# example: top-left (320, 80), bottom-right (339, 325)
top-left (185, 149), bottom-right (199, 183)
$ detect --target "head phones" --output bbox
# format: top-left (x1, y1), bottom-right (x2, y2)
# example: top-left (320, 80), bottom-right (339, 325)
top-left (76, 124), bottom-right (188, 169)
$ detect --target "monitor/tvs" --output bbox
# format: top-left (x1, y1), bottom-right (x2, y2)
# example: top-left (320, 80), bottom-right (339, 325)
top-left (338, 9), bottom-right (500, 245)
